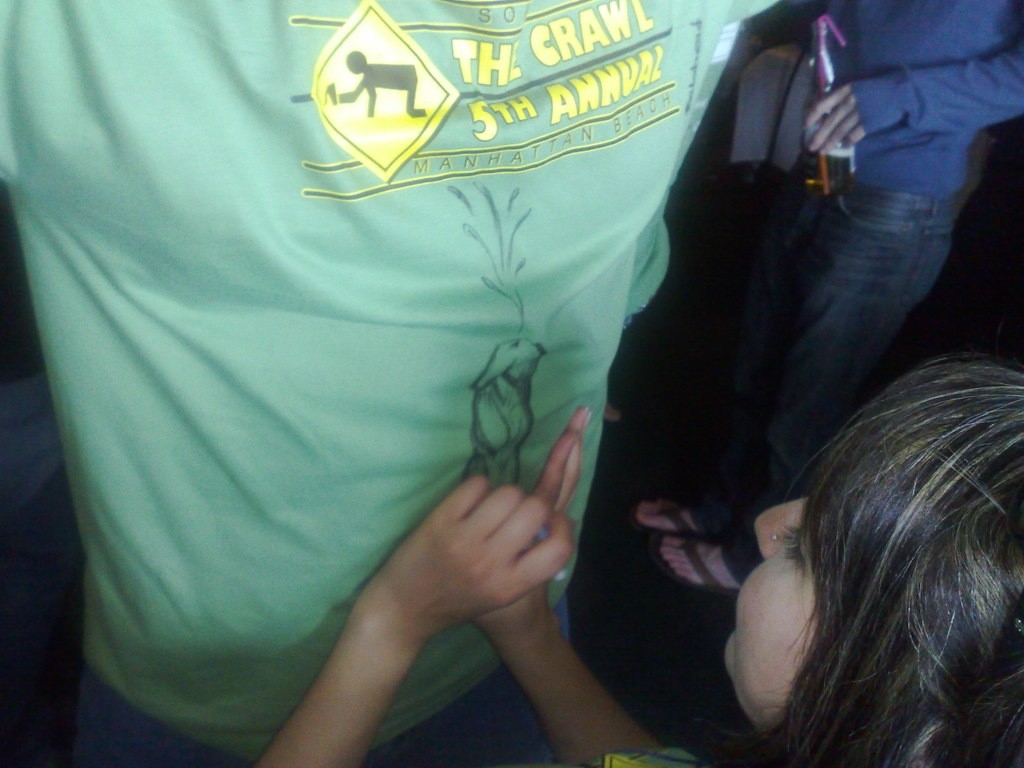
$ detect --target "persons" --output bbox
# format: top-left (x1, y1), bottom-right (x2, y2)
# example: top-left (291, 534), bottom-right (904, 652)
top-left (630, 0), bottom-right (1024, 595)
top-left (253, 351), bottom-right (1024, 768)
top-left (1, 0), bottom-right (791, 767)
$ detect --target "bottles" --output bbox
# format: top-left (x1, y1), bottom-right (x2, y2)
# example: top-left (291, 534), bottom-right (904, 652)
top-left (804, 22), bottom-right (855, 196)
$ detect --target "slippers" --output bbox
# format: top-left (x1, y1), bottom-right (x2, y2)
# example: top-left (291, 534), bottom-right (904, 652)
top-left (655, 497), bottom-right (741, 595)
